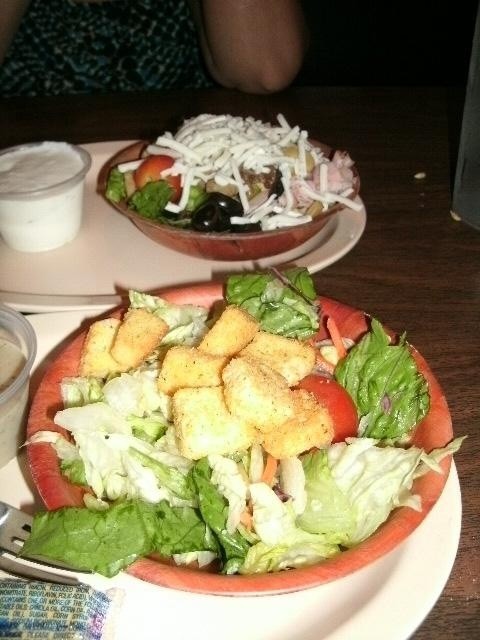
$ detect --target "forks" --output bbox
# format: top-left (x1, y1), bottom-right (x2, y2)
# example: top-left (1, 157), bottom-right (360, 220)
top-left (0, 501), bottom-right (93, 588)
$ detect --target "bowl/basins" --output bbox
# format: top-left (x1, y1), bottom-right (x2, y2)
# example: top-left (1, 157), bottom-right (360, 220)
top-left (26, 284), bottom-right (455, 600)
top-left (97, 134), bottom-right (361, 263)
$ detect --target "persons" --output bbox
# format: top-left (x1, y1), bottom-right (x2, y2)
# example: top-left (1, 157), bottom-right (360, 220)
top-left (1, 1), bottom-right (307, 94)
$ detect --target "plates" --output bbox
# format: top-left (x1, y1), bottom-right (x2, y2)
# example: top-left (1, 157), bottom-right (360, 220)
top-left (0, 313), bottom-right (463, 639)
top-left (0, 139), bottom-right (367, 315)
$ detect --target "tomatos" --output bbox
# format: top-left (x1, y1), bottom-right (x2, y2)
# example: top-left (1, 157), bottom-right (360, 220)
top-left (133, 155), bottom-right (181, 203)
